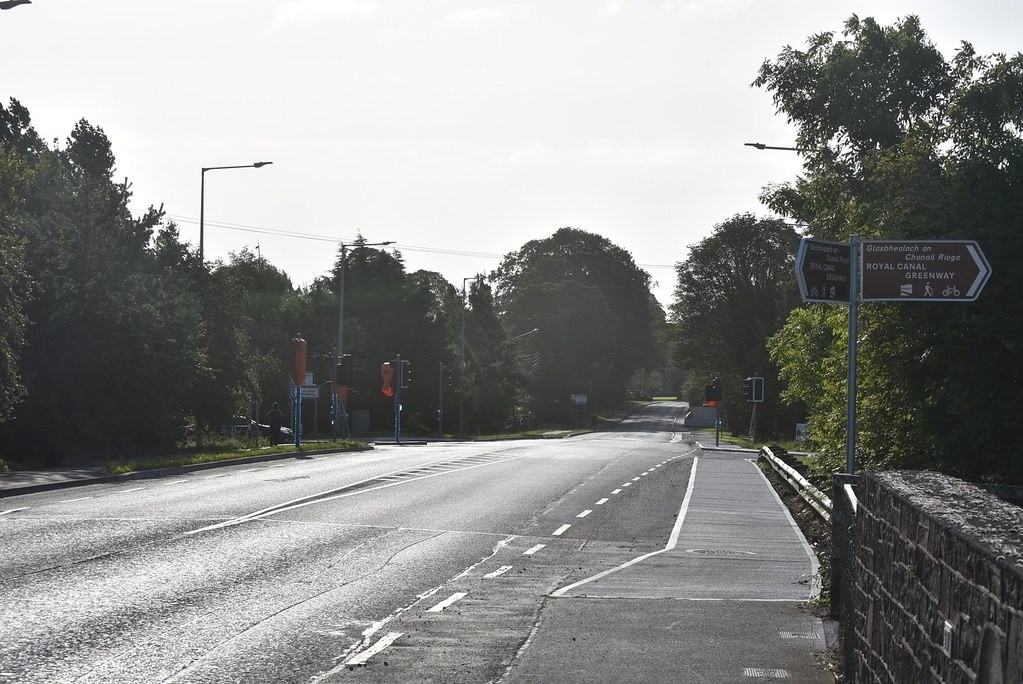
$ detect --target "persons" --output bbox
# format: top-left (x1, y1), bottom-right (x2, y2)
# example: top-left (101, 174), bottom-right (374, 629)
top-left (265, 402), bottom-right (283, 446)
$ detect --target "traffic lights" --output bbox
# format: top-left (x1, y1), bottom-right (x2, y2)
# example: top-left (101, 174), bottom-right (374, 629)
top-left (704, 377), bottom-right (722, 402)
top-left (389, 360), bottom-right (398, 393)
top-left (442, 366), bottom-right (453, 393)
top-left (312, 355), bottom-right (325, 385)
top-left (742, 376), bottom-right (764, 403)
top-left (401, 360), bottom-right (415, 389)
top-left (343, 354), bottom-right (354, 387)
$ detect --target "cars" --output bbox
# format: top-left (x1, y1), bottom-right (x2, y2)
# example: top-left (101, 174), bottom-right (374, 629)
top-left (185, 416), bottom-right (294, 444)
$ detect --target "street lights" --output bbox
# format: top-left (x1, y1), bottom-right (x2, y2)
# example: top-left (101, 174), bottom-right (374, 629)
top-left (197, 161), bottom-right (274, 452)
top-left (459, 275), bottom-right (498, 436)
top-left (334, 241), bottom-right (397, 441)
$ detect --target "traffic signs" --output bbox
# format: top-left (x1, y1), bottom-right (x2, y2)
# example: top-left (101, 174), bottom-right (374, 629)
top-left (860, 241), bottom-right (992, 301)
top-left (795, 238), bottom-right (852, 303)
